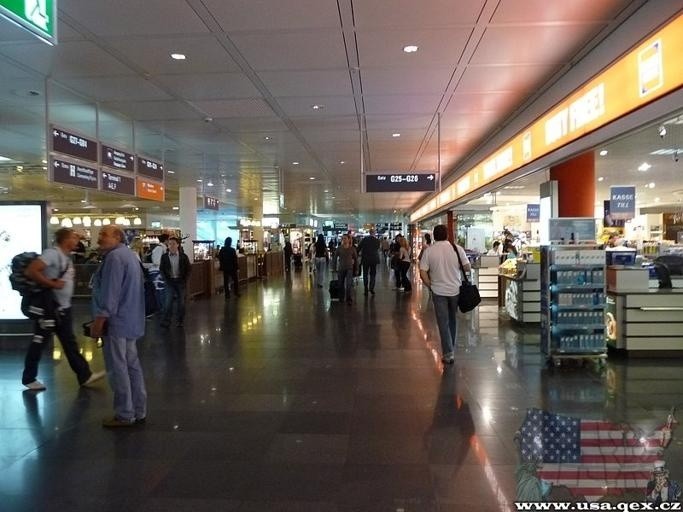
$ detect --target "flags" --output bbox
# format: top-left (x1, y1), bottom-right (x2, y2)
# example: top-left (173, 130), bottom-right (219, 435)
top-left (519, 409), bottom-right (672, 498)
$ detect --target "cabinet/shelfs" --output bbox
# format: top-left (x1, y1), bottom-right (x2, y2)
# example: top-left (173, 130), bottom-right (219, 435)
top-left (539, 245), bottom-right (608, 361)
top-left (540, 369), bottom-right (608, 424)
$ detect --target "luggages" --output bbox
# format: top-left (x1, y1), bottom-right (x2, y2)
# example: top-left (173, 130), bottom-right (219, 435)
top-left (329, 270), bottom-right (339, 300)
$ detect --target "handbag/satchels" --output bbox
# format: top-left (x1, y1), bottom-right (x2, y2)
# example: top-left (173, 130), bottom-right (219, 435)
top-left (8, 251), bottom-right (44, 298)
top-left (458, 280), bottom-right (482, 313)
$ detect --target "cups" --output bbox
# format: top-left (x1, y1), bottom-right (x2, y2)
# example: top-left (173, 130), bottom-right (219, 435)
top-left (604, 214), bottom-right (614, 227)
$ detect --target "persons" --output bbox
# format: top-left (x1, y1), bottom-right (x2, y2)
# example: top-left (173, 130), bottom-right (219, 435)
top-left (425, 361), bottom-right (475, 511)
top-left (23, 229), bottom-right (517, 426)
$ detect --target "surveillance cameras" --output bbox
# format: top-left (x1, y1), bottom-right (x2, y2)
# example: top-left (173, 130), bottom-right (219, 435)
top-left (674, 156), bottom-right (679, 162)
top-left (660, 129), bottom-right (666, 138)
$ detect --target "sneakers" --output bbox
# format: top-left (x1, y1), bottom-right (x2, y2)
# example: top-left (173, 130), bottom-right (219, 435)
top-left (81, 369), bottom-right (107, 387)
top-left (101, 413), bottom-right (135, 426)
top-left (441, 355), bottom-right (455, 365)
top-left (22, 379), bottom-right (47, 391)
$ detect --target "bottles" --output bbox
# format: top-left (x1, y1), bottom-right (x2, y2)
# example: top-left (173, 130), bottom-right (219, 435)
top-left (642, 241), bottom-right (662, 256)
